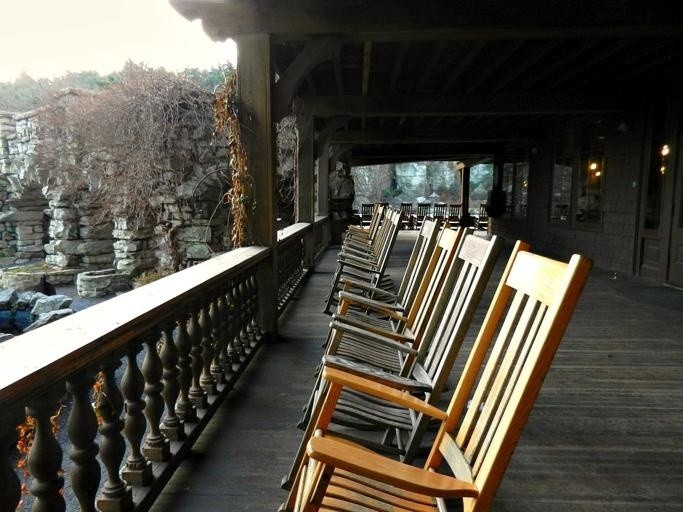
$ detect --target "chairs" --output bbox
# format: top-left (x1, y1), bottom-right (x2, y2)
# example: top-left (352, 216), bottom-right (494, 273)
top-left (334, 210), bottom-right (393, 275)
top-left (321, 215), bottom-right (401, 317)
top-left (474, 203), bottom-right (489, 229)
top-left (279, 240), bottom-right (590, 512)
top-left (336, 209), bottom-right (390, 271)
top-left (400, 203), bottom-right (414, 230)
top-left (348, 206), bottom-right (376, 237)
top-left (344, 204), bottom-right (379, 246)
top-left (449, 203), bottom-right (462, 229)
top-left (345, 203), bottom-right (375, 241)
top-left (416, 203), bottom-right (432, 230)
top-left (314, 216), bottom-right (440, 371)
top-left (342, 206), bottom-right (384, 252)
top-left (324, 211), bottom-right (398, 303)
top-left (376, 203), bottom-right (389, 224)
top-left (274, 227), bottom-right (500, 509)
top-left (434, 203), bottom-right (447, 229)
top-left (339, 207), bottom-right (384, 259)
top-left (280, 223), bottom-right (465, 489)
